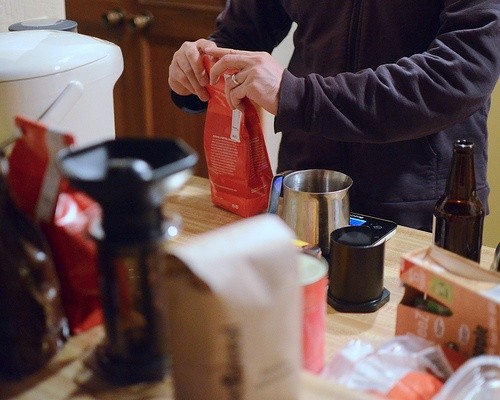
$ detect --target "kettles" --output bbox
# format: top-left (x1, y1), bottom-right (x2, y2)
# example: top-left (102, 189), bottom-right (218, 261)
top-left (0, 30), bottom-right (124, 166)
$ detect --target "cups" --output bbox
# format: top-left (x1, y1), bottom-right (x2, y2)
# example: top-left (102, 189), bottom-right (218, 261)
top-left (331, 225), bottom-right (386, 306)
top-left (7, 17), bottom-right (78, 32)
top-left (99, 156), bottom-right (167, 379)
top-left (303, 251), bottom-right (329, 375)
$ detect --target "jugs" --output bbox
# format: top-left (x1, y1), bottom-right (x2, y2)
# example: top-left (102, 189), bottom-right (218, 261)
top-left (267, 169), bottom-right (353, 255)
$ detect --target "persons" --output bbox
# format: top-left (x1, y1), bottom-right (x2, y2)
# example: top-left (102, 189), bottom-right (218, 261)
top-left (168, 0), bottom-right (500, 233)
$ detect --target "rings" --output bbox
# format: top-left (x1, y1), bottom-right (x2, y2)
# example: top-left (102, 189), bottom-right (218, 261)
top-left (232, 73), bottom-right (241, 86)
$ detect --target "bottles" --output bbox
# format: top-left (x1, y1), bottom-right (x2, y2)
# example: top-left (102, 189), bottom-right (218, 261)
top-left (433, 140), bottom-right (485, 265)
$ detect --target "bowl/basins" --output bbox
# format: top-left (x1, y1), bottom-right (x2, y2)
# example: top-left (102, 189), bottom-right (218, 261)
top-left (54, 135), bottom-right (200, 206)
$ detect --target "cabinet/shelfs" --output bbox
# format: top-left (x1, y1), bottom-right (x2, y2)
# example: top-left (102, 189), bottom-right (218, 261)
top-left (63, 0), bottom-right (229, 176)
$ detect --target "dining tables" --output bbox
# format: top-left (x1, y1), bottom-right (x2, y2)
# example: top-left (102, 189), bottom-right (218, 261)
top-left (0, 174), bottom-right (496, 400)
